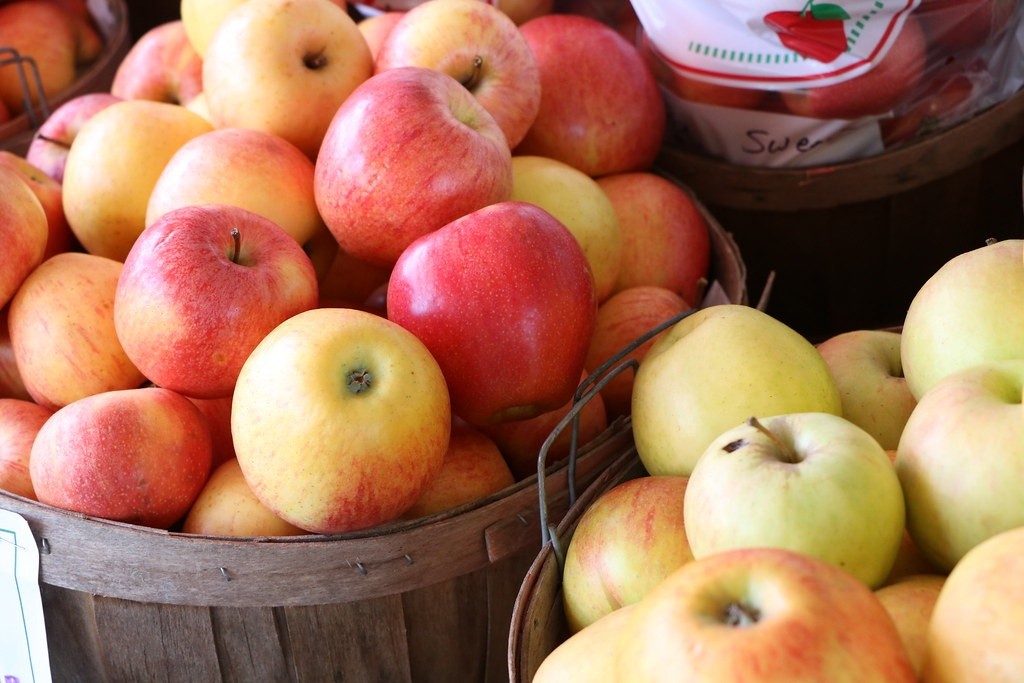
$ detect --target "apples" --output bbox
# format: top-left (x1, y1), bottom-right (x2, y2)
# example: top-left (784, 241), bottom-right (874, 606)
top-left (0, 0), bottom-right (713, 540)
top-left (624, 0), bottom-right (1024, 161)
top-left (525, 237), bottom-right (1024, 683)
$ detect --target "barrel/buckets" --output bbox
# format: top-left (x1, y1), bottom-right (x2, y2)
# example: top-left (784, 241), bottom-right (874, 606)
top-left (0, 0), bottom-right (1024, 683)
